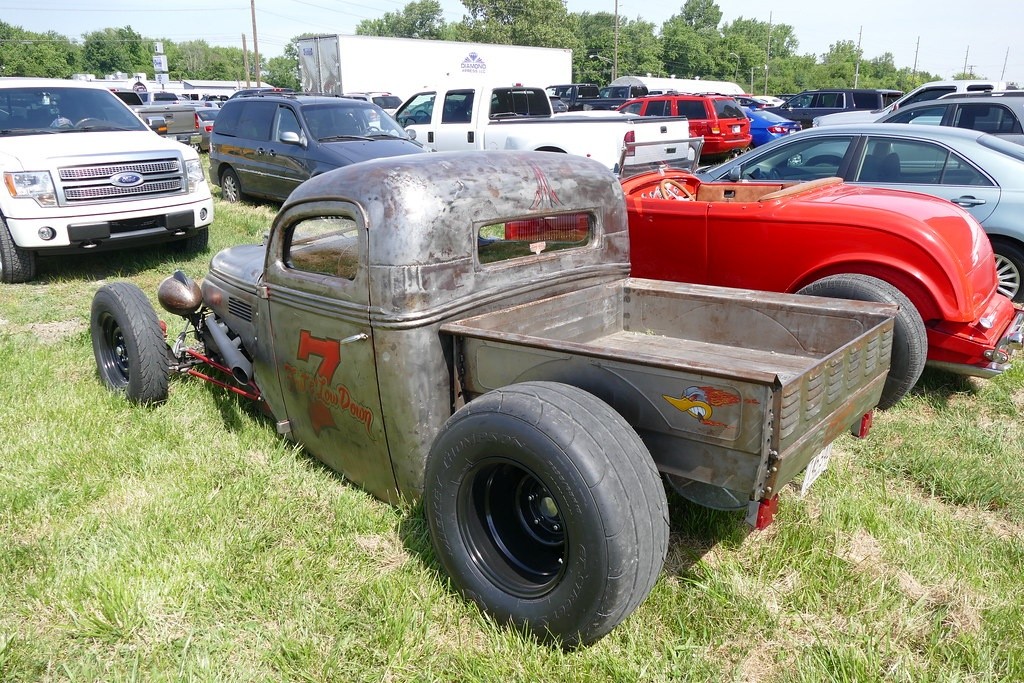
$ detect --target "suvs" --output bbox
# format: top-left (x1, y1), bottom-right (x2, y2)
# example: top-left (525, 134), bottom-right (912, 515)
top-left (613, 93), bottom-right (752, 164)
top-left (600, 77), bottom-right (1023, 176)
top-left (210, 95), bottom-right (430, 205)
top-left (0, 77), bottom-right (213, 285)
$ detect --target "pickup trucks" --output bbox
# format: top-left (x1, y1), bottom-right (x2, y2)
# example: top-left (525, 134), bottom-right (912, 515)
top-left (549, 82), bottom-right (633, 112)
top-left (90, 149), bottom-right (899, 653)
top-left (368, 82), bottom-right (695, 177)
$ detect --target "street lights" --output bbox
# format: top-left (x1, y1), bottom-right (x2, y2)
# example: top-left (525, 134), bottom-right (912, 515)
top-left (729, 52), bottom-right (740, 82)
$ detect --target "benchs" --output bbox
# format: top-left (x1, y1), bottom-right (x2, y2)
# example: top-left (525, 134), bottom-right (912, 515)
top-left (756, 176), bottom-right (843, 204)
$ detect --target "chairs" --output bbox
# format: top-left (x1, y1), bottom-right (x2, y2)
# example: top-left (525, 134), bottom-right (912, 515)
top-left (861, 153), bottom-right (883, 181)
top-left (885, 152), bottom-right (901, 183)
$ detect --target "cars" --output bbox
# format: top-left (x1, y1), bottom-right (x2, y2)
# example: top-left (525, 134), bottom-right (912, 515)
top-left (507, 132), bottom-right (1023, 412)
top-left (693, 124), bottom-right (1023, 307)
